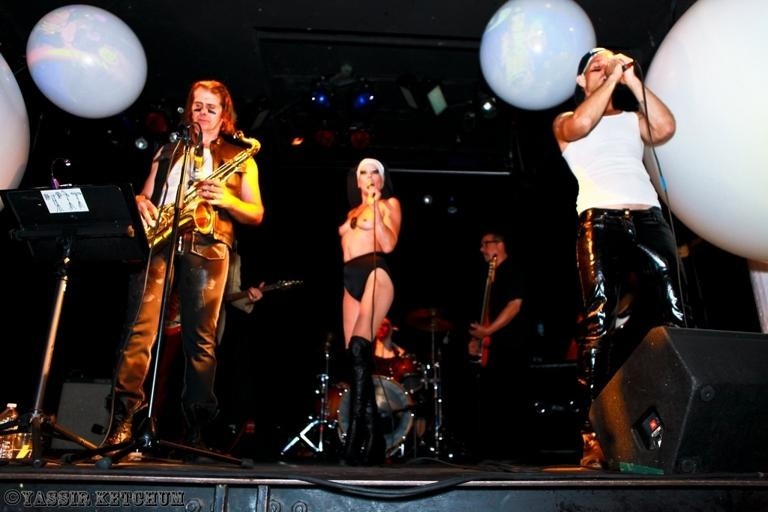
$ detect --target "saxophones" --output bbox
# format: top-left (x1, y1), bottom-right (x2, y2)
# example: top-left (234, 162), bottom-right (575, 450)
top-left (146, 129), bottom-right (261, 249)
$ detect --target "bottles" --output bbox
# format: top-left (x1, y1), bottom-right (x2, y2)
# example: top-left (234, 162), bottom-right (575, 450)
top-left (0, 403), bottom-right (18, 461)
top-left (18, 433), bottom-right (31, 462)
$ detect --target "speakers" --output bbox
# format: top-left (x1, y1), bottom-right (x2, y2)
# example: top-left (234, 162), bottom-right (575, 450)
top-left (588, 326), bottom-right (768, 479)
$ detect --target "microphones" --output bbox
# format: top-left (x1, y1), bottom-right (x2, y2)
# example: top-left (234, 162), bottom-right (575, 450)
top-left (622, 60), bottom-right (638, 72)
top-left (367, 180), bottom-right (375, 197)
top-left (194, 130), bottom-right (203, 168)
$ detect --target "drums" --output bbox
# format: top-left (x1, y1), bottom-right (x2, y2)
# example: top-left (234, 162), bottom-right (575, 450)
top-left (380, 353), bottom-right (423, 395)
top-left (316, 372), bottom-right (413, 459)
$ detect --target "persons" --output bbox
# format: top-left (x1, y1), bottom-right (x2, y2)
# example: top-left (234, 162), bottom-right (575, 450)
top-left (553, 47), bottom-right (692, 468)
top-left (102, 79), bottom-right (264, 467)
top-left (338, 157), bottom-right (402, 467)
top-left (469, 233), bottom-right (526, 467)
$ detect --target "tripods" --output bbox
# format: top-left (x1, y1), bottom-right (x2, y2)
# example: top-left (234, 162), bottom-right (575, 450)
top-left (281, 331), bottom-right (338, 462)
top-left (0, 218), bottom-right (98, 465)
top-left (59, 144), bottom-right (254, 469)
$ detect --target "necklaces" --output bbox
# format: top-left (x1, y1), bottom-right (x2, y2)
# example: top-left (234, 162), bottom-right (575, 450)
top-left (350, 204), bottom-right (368, 229)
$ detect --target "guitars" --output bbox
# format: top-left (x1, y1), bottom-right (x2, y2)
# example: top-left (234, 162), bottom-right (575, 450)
top-left (468, 253), bottom-right (498, 368)
top-left (159, 275), bottom-right (304, 338)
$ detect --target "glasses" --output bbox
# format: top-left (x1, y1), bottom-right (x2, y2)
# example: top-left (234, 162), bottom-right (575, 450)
top-left (480, 239), bottom-right (499, 246)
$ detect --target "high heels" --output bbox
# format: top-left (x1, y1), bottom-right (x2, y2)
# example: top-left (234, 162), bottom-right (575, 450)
top-left (339, 335), bottom-right (387, 467)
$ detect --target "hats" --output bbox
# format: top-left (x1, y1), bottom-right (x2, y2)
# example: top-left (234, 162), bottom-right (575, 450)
top-left (577, 47), bottom-right (608, 75)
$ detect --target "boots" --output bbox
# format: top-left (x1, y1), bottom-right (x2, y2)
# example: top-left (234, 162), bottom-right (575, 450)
top-left (169, 403), bottom-right (221, 461)
top-left (94, 386), bottom-right (148, 459)
